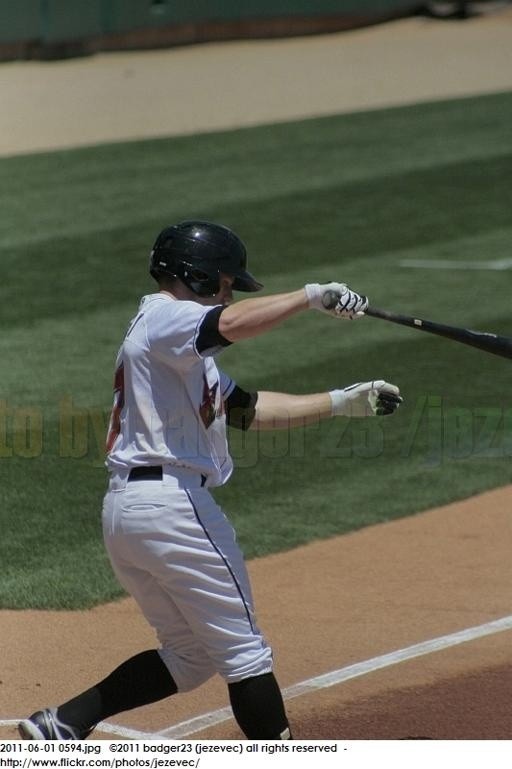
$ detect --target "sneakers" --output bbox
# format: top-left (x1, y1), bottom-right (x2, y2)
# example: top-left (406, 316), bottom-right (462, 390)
top-left (17, 703), bottom-right (83, 740)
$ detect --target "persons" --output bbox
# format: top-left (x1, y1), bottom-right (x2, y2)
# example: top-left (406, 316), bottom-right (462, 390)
top-left (17, 221), bottom-right (403, 740)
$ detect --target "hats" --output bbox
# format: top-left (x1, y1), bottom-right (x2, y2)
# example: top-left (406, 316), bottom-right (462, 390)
top-left (148, 219), bottom-right (265, 300)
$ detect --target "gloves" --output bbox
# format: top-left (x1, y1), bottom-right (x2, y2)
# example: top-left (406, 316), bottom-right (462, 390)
top-left (327, 379), bottom-right (404, 419)
top-left (304, 280), bottom-right (372, 322)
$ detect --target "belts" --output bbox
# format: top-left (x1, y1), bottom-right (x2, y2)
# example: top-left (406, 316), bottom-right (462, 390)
top-left (128, 464), bottom-right (209, 488)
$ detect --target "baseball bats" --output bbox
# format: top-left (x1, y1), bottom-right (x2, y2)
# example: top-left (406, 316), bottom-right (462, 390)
top-left (319, 289), bottom-right (512, 360)
